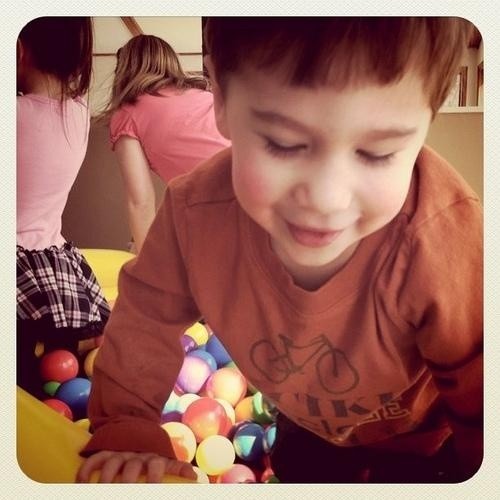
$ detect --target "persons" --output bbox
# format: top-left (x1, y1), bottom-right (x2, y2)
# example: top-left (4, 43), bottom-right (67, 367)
top-left (76, 16), bottom-right (483, 484)
top-left (17, 16), bottom-right (114, 362)
top-left (98, 35), bottom-right (234, 254)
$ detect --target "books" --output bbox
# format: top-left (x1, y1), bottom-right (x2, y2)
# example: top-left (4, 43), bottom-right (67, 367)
top-left (457, 64), bottom-right (480, 107)
